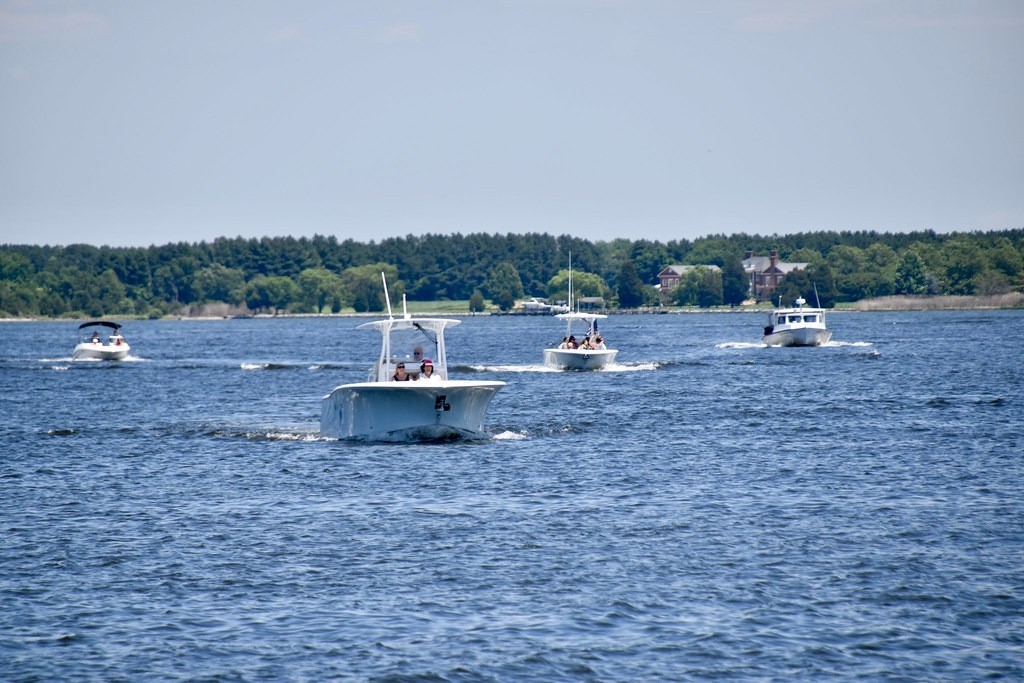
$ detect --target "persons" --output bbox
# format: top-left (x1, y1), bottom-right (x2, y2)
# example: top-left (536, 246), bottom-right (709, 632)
top-left (392, 361), bottom-right (415, 382)
top-left (90, 331), bottom-right (100, 343)
top-left (416, 359), bottom-right (441, 382)
top-left (110, 331), bottom-right (122, 345)
top-left (560, 334), bottom-right (607, 352)
top-left (413, 345), bottom-right (424, 362)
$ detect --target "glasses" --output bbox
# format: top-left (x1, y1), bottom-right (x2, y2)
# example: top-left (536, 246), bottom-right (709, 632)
top-left (397, 365), bottom-right (405, 369)
top-left (414, 352), bottom-right (420, 355)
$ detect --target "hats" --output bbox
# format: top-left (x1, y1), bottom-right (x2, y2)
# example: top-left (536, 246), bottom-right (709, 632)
top-left (423, 360), bottom-right (433, 365)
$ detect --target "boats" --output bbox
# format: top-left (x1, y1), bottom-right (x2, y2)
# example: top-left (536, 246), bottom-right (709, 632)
top-left (74, 321), bottom-right (131, 359)
top-left (761, 281), bottom-right (834, 348)
top-left (319, 272), bottom-right (508, 441)
top-left (542, 249), bottom-right (619, 370)
